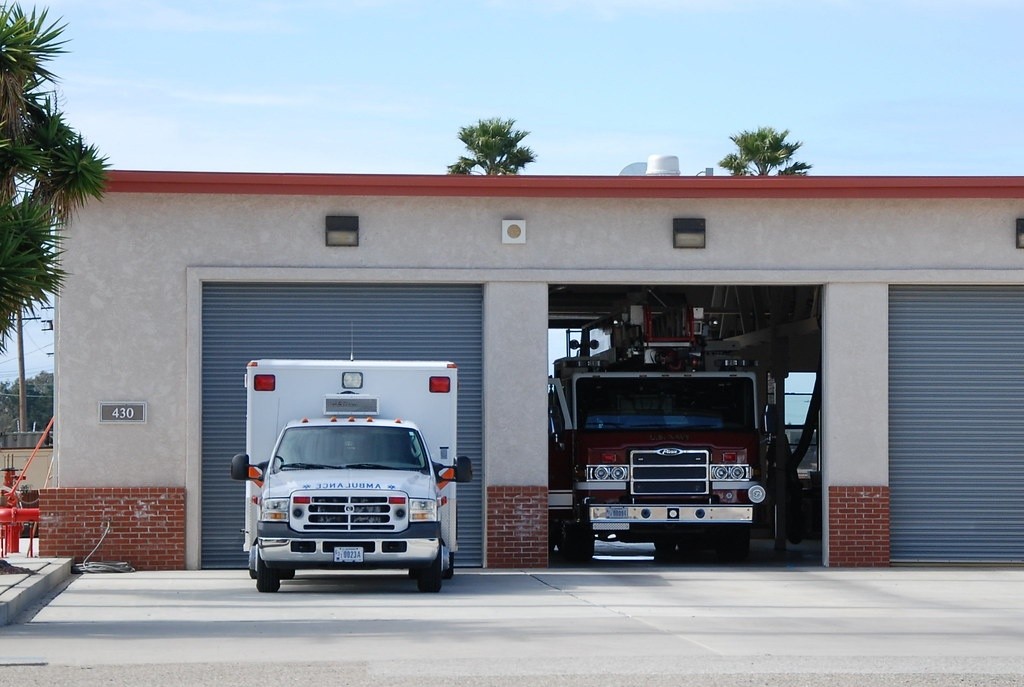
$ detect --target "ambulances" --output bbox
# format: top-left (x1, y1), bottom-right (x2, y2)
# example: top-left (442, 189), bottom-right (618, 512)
top-left (231, 359), bottom-right (474, 594)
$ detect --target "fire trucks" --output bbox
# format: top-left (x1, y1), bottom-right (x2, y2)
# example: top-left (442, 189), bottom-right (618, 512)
top-left (545, 284), bottom-right (782, 563)
